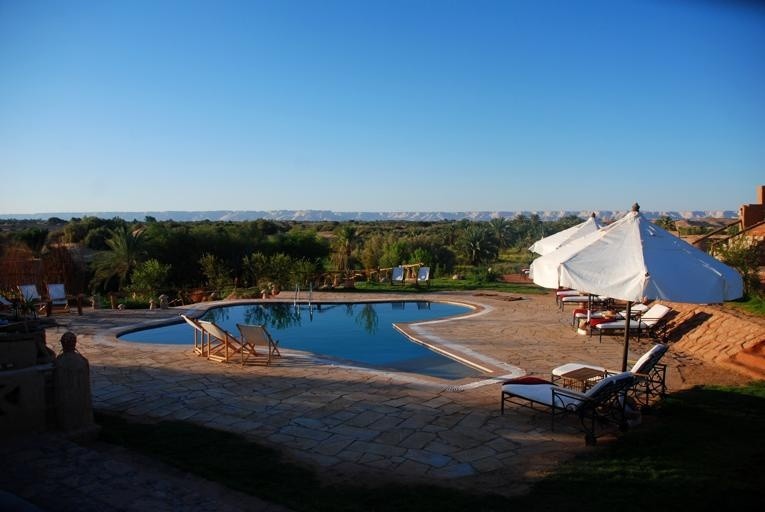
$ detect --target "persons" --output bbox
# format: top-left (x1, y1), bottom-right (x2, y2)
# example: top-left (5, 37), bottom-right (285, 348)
top-left (610, 308), bottom-right (616, 315)
top-left (601, 306), bottom-right (612, 319)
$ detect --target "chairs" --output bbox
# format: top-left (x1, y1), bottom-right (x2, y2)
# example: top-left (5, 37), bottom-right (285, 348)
top-left (554, 288), bottom-right (672, 341)
top-left (500, 371), bottom-right (649, 448)
top-left (0, 283), bottom-right (71, 318)
top-left (551, 343), bottom-right (669, 431)
top-left (390, 266), bottom-right (405, 287)
top-left (178, 313), bottom-right (280, 367)
top-left (416, 266), bottom-right (431, 288)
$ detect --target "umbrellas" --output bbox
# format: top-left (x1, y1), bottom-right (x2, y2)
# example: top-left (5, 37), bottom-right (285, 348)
top-left (527, 213), bottom-right (609, 323)
top-left (529, 203), bottom-right (742, 409)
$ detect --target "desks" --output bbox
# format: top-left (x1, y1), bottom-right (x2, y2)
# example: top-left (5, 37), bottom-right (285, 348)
top-left (560, 367), bottom-right (608, 392)
top-left (404, 277), bottom-right (417, 284)
